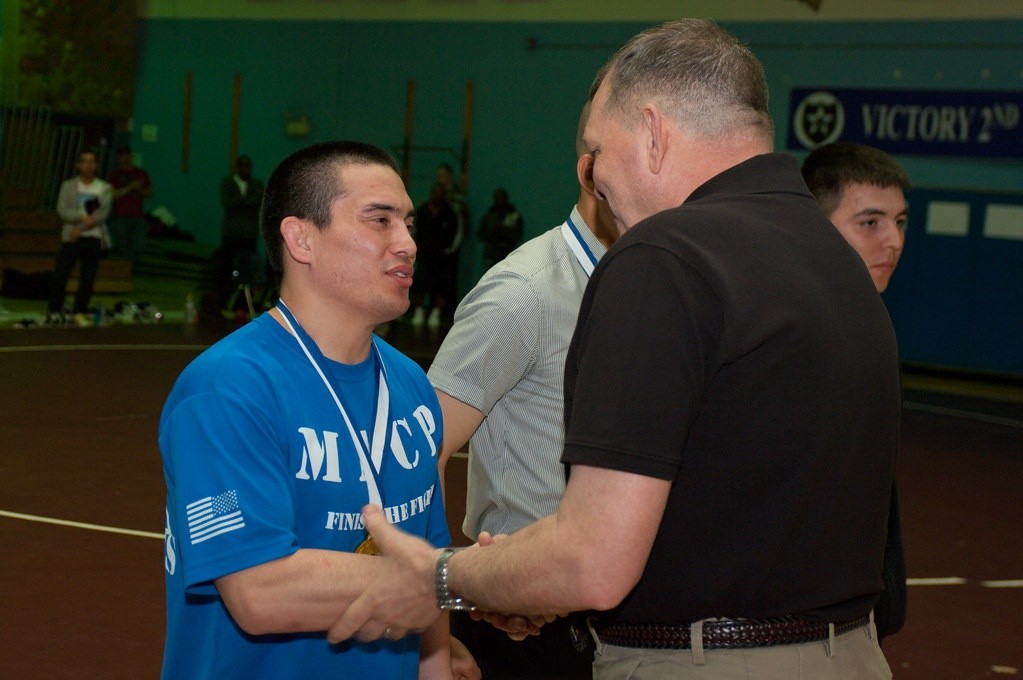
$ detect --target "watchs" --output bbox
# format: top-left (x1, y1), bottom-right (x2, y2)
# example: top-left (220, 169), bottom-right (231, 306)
top-left (436, 546), bottom-right (481, 611)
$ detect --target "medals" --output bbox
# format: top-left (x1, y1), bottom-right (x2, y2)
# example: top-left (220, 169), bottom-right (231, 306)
top-left (355, 537), bottom-right (381, 556)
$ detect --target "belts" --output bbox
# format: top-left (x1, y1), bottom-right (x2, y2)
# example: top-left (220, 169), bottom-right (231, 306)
top-left (593, 610), bottom-right (869, 649)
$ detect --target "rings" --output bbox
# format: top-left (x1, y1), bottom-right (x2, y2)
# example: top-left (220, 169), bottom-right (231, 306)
top-left (386, 629), bottom-right (390, 637)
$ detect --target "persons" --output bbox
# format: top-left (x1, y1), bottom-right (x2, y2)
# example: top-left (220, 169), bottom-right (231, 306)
top-left (106, 149), bottom-right (153, 273)
top-left (475, 187), bottom-right (523, 274)
top-left (50, 152), bottom-right (113, 315)
top-left (158, 142), bottom-right (566, 680)
top-left (400, 164), bottom-right (469, 328)
top-left (423, 73), bottom-right (622, 679)
top-left (803, 141), bottom-right (909, 292)
top-left (329, 19), bottom-right (904, 680)
top-left (217, 153), bottom-right (263, 266)
